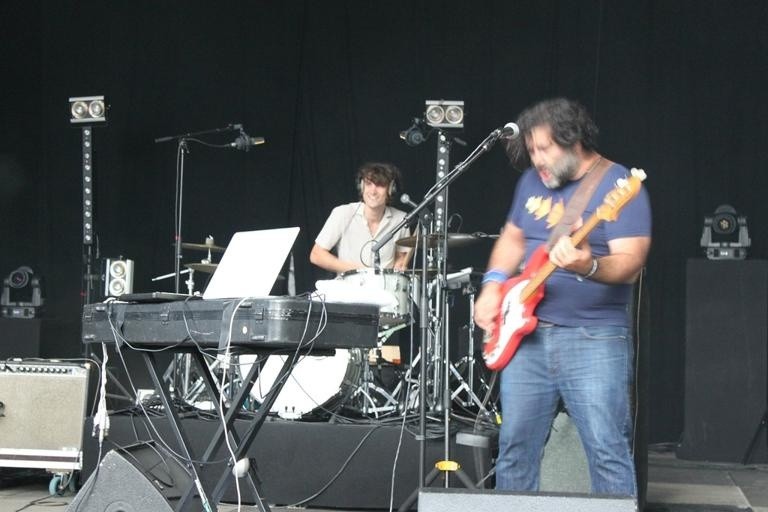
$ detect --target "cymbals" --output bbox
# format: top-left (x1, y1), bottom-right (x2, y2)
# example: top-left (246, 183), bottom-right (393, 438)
top-left (404, 268), bottom-right (464, 278)
top-left (185, 264), bottom-right (217, 273)
top-left (278, 274), bottom-right (286, 279)
top-left (396, 233), bottom-right (476, 248)
top-left (172, 243), bottom-right (226, 253)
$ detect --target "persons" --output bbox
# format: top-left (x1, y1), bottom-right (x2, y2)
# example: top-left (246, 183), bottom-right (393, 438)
top-left (310, 162), bottom-right (411, 275)
top-left (472, 98), bottom-right (652, 501)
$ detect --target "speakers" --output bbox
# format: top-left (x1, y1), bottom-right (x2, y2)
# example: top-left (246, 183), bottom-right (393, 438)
top-left (699, 204), bottom-right (752, 259)
top-left (66, 439), bottom-right (194, 512)
top-left (105, 257), bottom-right (134, 298)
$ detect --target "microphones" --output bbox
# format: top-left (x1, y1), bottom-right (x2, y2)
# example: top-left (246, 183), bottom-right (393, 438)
top-left (400, 194), bottom-right (417, 209)
top-left (235, 135), bottom-right (266, 149)
top-left (398, 130), bottom-right (424, 148)
top-left (495, 122), bottom-right (521, 141)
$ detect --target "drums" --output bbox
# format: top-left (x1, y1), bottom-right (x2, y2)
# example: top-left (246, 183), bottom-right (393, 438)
top-left (340, 268), bottom-right (410, 323)
top-left (237, 348), bottom-right (363, 420)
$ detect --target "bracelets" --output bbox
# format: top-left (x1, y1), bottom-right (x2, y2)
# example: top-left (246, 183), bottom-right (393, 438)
top-left (576, 259), bottom-right (598, 283)
top-left (480, 270), bottom-right (508, 284)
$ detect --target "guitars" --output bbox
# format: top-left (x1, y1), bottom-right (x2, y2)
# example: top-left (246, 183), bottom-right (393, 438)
top-left (482, 168), bottom-right (647, 369)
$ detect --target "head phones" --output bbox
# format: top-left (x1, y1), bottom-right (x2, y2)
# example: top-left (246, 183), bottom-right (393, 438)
top-left (356, 160), bottom-right (401, 201)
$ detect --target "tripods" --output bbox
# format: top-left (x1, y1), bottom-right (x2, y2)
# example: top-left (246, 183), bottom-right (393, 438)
top-left (157, 351), bottom-right (229, 406)
top-left (343, 348), bottom-right (401, 416)
top-left (378, 290), bottom-right (496, 423)
